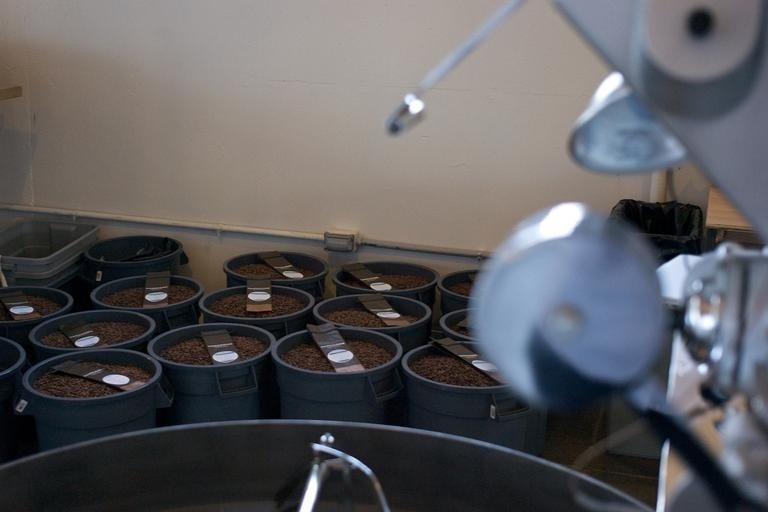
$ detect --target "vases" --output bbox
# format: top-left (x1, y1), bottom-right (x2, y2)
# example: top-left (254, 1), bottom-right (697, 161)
top-left (84, 234), bottom-right (190, 287)
top-left (441, 304), bottom-right (481, 342)
top-left (89, 274), bottom-right (204, 335)
top-left (1, 281), bottom-right (73, 332)
top-left (271, 326), bottom-right (404, 423)
top-left (405, 339), bottom-right (536, 453)
top-left (313, 293), bottom-right (434, 349)
top-left (1, 336), bottom-right (28, 382)
top-left (26, 308), bottom-right (157, 356)
top-left (221, 249), bottom-right (330, 305)
top-left (436, 266), bottom-right (486, 306)
top-left (20, 349), bottom-right (176, 452)
top-left (331, 260), bottom-right (438, 307)
top-left (198, 285), bottom-right (315, 336)
top-left (146, 321), bottom-right (279, 426)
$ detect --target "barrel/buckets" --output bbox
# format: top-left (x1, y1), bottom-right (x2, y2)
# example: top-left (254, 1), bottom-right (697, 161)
top-left (0, 235), bottom-right (549, 456)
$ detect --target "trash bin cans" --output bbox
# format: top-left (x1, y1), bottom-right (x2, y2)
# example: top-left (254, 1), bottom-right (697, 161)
top-left (601, 200), bottom-right (705, 260)
